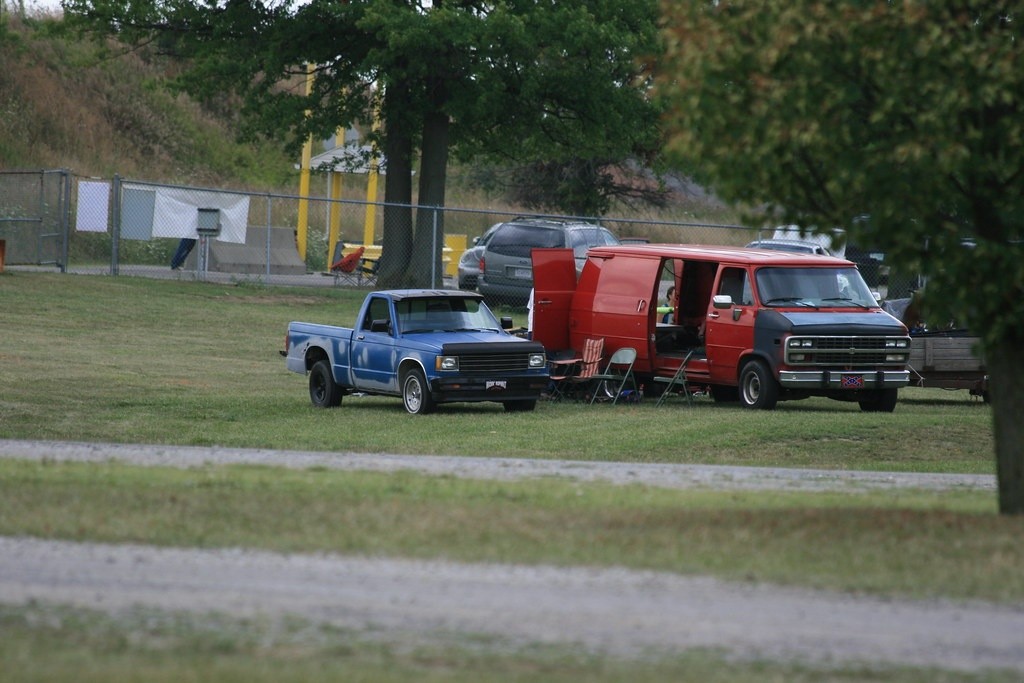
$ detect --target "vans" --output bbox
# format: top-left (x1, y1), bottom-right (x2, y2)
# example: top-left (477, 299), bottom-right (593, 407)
top-left (527, 239), bottom-right (912, 412)
top-left (738, 220), bottom-right (855, 300)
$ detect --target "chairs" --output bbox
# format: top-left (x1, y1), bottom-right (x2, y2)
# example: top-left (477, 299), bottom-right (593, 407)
top-left (550, 338), bottom-right (605, 403)
top-left (333, 246), bottom-right (382, 286)
top-left (652, 350), bottom-right (694, 410)
top-left (589, 348), bottom-right (637, 405)
top-left (970, 365), bottom-right (988, 403)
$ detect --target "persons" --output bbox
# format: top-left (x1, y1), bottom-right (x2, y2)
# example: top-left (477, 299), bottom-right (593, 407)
top-left (527, 288), bottom-right (534, 341)
top-left (657, 286), bottom-right (675, 324)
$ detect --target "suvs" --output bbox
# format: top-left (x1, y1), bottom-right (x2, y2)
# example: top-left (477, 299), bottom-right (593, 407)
top-left (456, 215), bottom-right (623, 312)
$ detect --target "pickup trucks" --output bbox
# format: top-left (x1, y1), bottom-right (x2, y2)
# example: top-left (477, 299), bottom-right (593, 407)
top-left (279, 287), bottom-right (550, 414)
